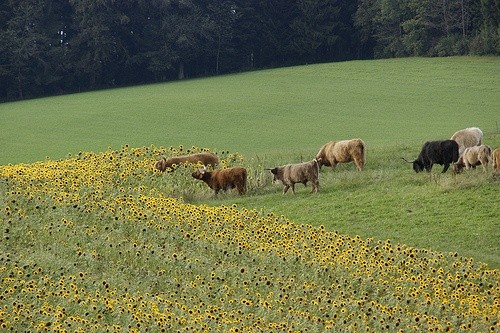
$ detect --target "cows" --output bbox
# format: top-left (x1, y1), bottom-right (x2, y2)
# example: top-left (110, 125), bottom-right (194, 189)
top-left (450, 127), bottom-right (484, 171)
top-left (491, 148), bottom-right (500, 171)
top-left (402, 140), bottom-right (459, 173)
top-left (454, 144), bottom-right (492, 174)
top-left (153, 154), bottom-right (219, 171)
top-left (192, 166), bottom-right (247, 196)
top-left (263, 158), bottom-right (322, 195)
top-left (314, 138), bottom-right (367, 172)
